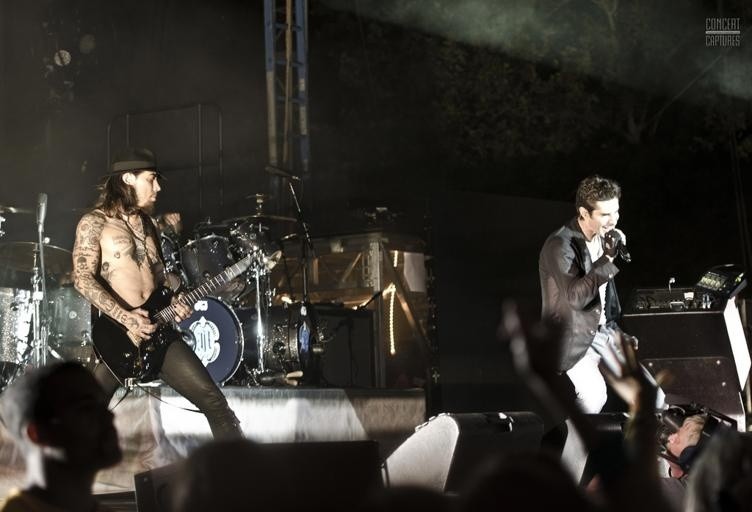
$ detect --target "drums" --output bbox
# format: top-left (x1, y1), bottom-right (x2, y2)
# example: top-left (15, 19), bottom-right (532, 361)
top-left (45, 282), bottom-right (98, 363)
top-left (0, 288), bottom-right (44, 368)
top-left (172, 294), bottom-right (245, 386)
top-left (242, 302), bottom-right (324, 381)
top-left (229, 222), bottom-right (281, 277)
top-left (179, 235), bottom-right (246, 302)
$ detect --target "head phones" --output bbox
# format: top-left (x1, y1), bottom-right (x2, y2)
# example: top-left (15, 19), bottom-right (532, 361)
top-left (680, 414), bottom-right (719, 474)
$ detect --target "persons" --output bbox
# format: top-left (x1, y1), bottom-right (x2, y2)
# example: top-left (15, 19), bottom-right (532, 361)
top-left (540, 176), bottom-right (667, 486)
top-left (72, 147), bottom-right (244, 442)
top-left (155, 211), bottom-right (183, 233)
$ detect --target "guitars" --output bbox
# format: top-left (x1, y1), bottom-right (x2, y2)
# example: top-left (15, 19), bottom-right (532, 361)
top-left (90, 252), bottom-right (258, 380)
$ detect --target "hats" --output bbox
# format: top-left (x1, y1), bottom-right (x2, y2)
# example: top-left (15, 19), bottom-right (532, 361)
top-left (97, 148), bottom-right (164, 183)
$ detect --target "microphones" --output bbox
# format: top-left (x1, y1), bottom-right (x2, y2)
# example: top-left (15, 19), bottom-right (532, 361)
top-left (655, 447), bottom-right (680, 465)
top-left (605, 225), bottom-right (632, 263)
top-left (43, 237), bottom-right (51, 243)
top-left (264, 165), bottom-right (301, 181)
top-left (36, 192), bottom-right (48, 225)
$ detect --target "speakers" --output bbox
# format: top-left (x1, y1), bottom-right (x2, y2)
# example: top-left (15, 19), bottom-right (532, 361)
top-left (134, 439), bottom-right (381, 512)
top-left (546, 413), bottom-right (623, 488)
top-left (382, 410), bottom-right (547, 496)
top-left (234, 309), bottom-right (375, 389)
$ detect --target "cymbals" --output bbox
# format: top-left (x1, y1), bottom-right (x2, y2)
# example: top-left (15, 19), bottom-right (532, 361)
top-left (223, 214), bottom-right (297, 224)
top-left (53, 273), bottom-right (75, 283)
top-left (1, 241), bottom-right (72, 273)
top-left (0, 205), bottom-right (37, 216)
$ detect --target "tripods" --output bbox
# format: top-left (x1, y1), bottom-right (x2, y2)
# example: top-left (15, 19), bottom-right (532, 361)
top-left (9, 264), bottom-right (75, 384)
top-left (237, 261), bottom-right (298, 387)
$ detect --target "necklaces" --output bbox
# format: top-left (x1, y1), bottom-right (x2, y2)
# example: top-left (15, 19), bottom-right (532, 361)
top-left (117, 212), bottom-right (154, 266)
